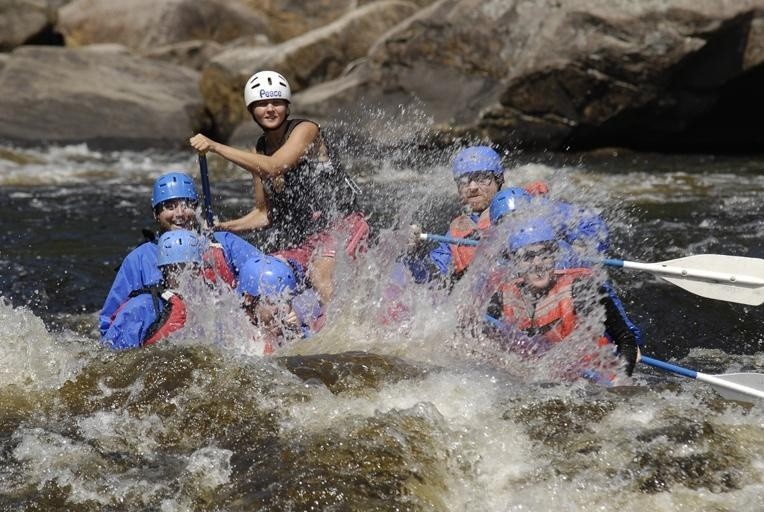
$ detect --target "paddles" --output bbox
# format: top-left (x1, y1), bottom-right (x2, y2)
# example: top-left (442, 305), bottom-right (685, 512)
top-left (640, 357), bottom-right (764, 409)
top-left (419, 233), bottom-right (760, 308)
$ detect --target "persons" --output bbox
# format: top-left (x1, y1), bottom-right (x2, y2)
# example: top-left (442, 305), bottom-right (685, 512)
top-left (98, 71), bottom-right (368, 352)
top-left (388, 147), bottom-right (642, 388)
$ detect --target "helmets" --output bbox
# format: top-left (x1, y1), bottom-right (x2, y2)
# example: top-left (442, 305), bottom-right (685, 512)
top-left (244, 70), bottom-right (292, 107)
top-left (156, 229), bottom-right (203, 266)
top-left (507, 219), bottom-right (556, 254)
top-left (452, 146), bottom-right (505, 182)
top-left (151, 172), bottom-right (200, 208)
top-left (488, 187), bottom-right (533, 225)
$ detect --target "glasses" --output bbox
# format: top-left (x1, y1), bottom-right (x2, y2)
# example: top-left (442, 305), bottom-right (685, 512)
top-left (518, 247), bottom-right (550, 263)
